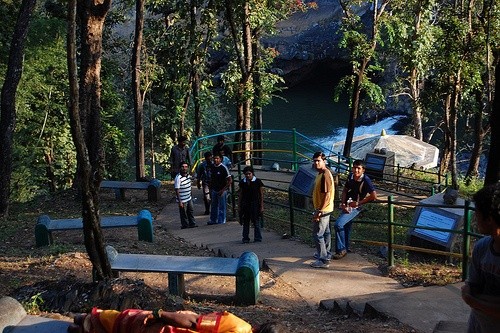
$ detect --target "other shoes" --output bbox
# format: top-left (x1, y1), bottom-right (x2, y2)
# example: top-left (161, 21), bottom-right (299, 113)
top-left (333, 250), bottom-right (347, 259)
top-left (347, 246), bottom-right (351, 251)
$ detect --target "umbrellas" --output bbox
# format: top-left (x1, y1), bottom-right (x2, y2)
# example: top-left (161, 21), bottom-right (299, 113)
top-left (333, 129), bottom-right (439, 169)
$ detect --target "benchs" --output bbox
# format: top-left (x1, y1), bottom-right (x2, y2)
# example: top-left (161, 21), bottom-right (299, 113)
top-left (0, 296), bottom-right (74, 333)
top-left (35, 210), bottom-right (152, 247)
top-left (100, 178), bottom-right (161, 203)
top-left (105, 245), bottom-right (260, 305)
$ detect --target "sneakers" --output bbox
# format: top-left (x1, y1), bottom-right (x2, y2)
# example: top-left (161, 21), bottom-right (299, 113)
top-left (310, 261), bottom-right (329, 267)
top-left (314, 251), bottom-right (331, 260)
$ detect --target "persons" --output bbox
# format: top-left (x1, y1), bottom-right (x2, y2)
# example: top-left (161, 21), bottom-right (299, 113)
top-left (67, 307), bottom-right (288, 333)
top-left (332, 159), bottom-right (377, 259)
top-left (169, 135), bottom-right (234, 229)
top-left (461, 184), bottom-right (500, 333)
top-left (238, 166), bottom-right (264, 243)
top-left (310, 152), bottom-right (335, 268)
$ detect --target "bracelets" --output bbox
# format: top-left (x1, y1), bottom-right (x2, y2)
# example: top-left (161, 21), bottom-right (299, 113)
top-left (315, 210), bottom-right (322, 217)
top-left (355, 200), bottom-right (360, 207)
top-left (153, 308), bottom-right (163, 319)
